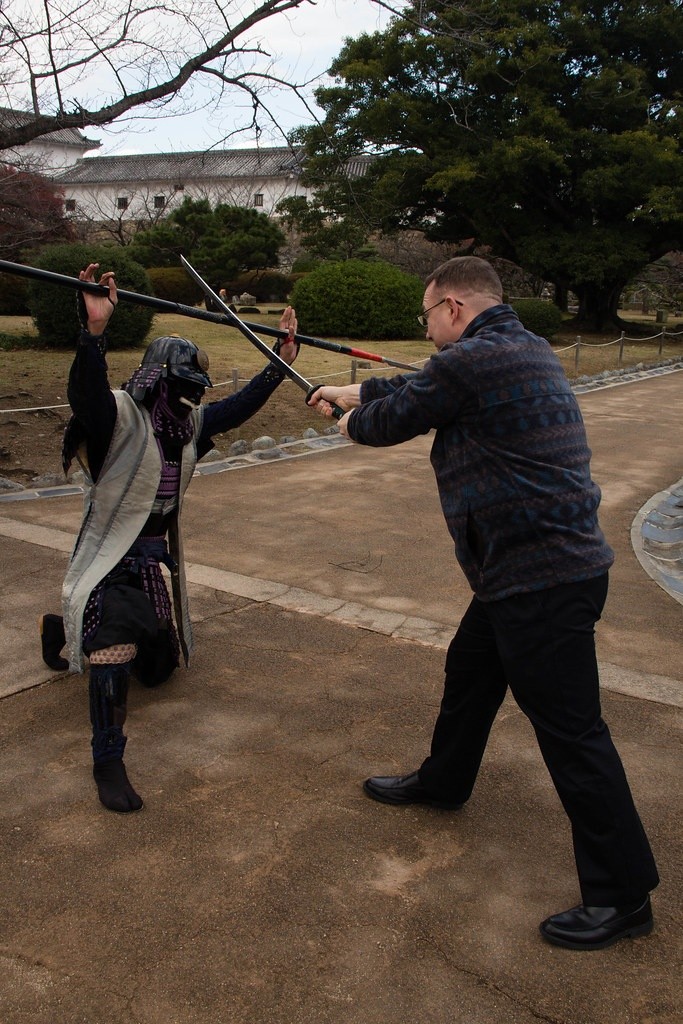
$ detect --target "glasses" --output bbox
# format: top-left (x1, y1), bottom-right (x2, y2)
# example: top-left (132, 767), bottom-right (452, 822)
top-left (416, 297), bottom-right (463, 327)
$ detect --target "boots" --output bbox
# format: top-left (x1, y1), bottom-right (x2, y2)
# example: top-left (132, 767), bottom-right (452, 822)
top-left (39, 613), bottom-right (71, 671)
top-left (90, 734), bottom-right (143, 813)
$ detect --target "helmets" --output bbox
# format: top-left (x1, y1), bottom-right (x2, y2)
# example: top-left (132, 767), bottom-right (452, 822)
top-left (120, 334), bottom-right (213, 402)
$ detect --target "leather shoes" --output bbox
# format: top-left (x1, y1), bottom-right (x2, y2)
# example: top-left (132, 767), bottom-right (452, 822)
top-left (538, 892), bottom-right (653, 950)
top-left (363, 769), bottom-right (463, 811)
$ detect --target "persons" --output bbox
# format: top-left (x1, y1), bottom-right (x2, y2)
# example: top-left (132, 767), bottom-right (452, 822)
top-left (41, 262), bottom-right (302, 815)
top-left (218, 289), bottom-right (227, 301)
top-left (308, 256), bottom-right (659, 951)
